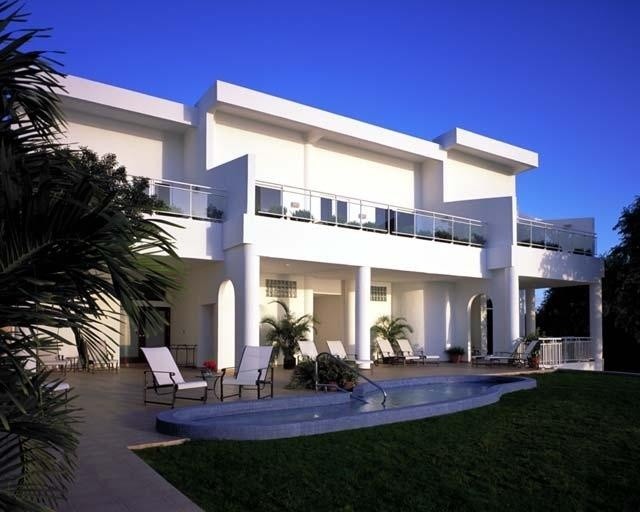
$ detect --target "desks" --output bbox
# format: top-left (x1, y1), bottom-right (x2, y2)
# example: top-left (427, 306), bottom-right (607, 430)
top-left (196, 375), bottom-right (220, 402)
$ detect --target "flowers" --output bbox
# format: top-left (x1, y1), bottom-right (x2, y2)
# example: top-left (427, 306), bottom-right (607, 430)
top-left (202, 358), bottom-right (218, 368)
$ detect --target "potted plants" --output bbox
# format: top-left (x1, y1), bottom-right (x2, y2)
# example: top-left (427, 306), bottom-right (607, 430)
top-left (371, 314), bottom-right (413, 365)
top-left (287, 354), bottom-right (359, 392)
top-left (526, 328), bottom-right (547, 369)
top-left (445, 346), bottom-right (465, 365)
top-left (261, 300), bottom-right (321, 370)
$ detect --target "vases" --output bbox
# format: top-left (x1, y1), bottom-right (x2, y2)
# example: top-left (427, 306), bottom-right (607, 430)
top-left (206, 368), bottom-right (217, 374)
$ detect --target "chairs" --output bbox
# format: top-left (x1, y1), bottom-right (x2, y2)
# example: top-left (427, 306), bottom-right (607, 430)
top-left (40, 353), bottom-right (120, 374)
top-left (219, 345), bottom-right (276, 404)
top-left (471, 336), bottom-right (523, 368)
top-left (378, 336), bottom-right (420, 368)
top-left (295, 339), bottom-right (320, 365)
top-left (141, 347), bottom-right (209, 410)
top-left (324, 340), bottom-right (374, 371)
top-left (396, 338), bottom-right (441, 368)
top-left (32, 379), bottom-right (72, 410)
top-left (487, 340), bottom-right (542, 369)
